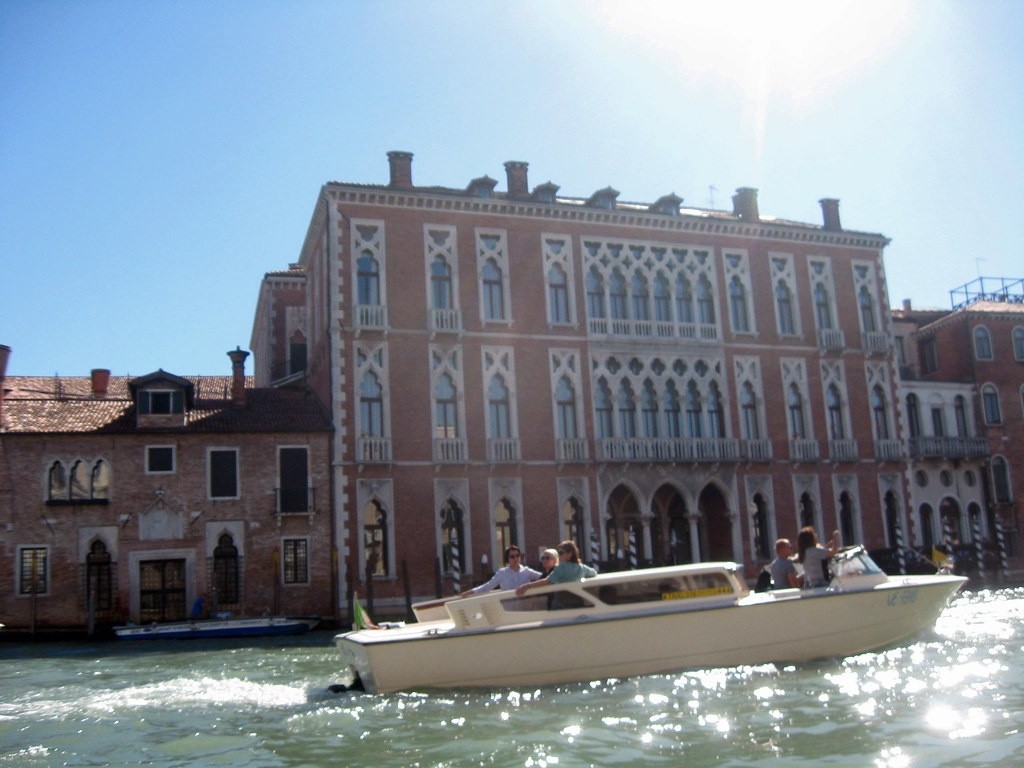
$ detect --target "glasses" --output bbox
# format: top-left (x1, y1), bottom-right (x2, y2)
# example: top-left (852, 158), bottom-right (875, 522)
top-left (510, 554), bottom-right (520, 558)
top-left (558, 551), bottom-right (567, 556)
top-left (541, 555), bottom-right (550, 561)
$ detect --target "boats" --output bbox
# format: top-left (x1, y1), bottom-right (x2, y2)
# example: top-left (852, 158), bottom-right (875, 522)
top-left (332, 559), bottom-right (969, 696)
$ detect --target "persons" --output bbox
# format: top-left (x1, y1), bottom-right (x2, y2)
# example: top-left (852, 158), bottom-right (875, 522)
top-left (460, 527), bottom-right (840, 610)
top-left (191, 593), bottom-right (207, 620)
